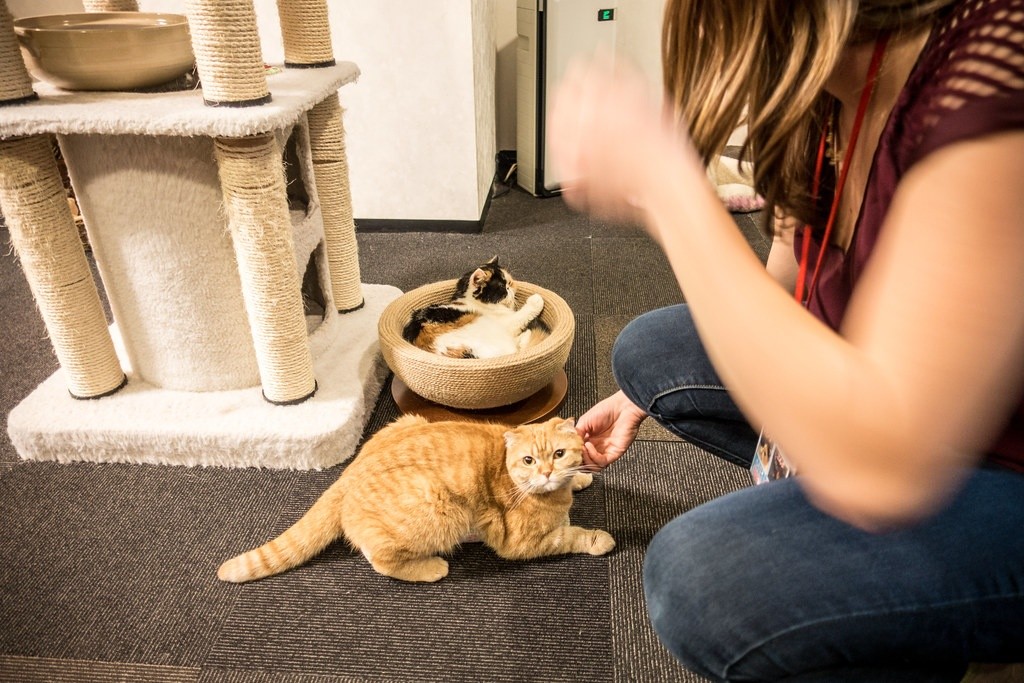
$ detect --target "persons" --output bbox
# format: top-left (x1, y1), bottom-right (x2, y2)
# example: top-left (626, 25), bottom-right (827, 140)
top-left (551, 0), bottom-right (1024, 683)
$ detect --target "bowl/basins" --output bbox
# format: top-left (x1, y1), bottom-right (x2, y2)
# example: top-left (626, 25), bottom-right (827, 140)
top-left (13, 11), bottom-right (195, 90)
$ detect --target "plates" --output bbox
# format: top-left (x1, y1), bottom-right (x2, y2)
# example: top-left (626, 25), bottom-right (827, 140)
top-left (392, 368), bottom-right (568, 427)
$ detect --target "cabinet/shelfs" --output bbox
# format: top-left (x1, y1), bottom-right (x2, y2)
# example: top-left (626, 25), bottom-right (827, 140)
top-left (0, 1), bottom-right (402, 474)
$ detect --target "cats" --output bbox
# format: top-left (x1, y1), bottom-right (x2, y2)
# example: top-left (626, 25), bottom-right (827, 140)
top-left (215, 411), bottom-right (615, 584)
